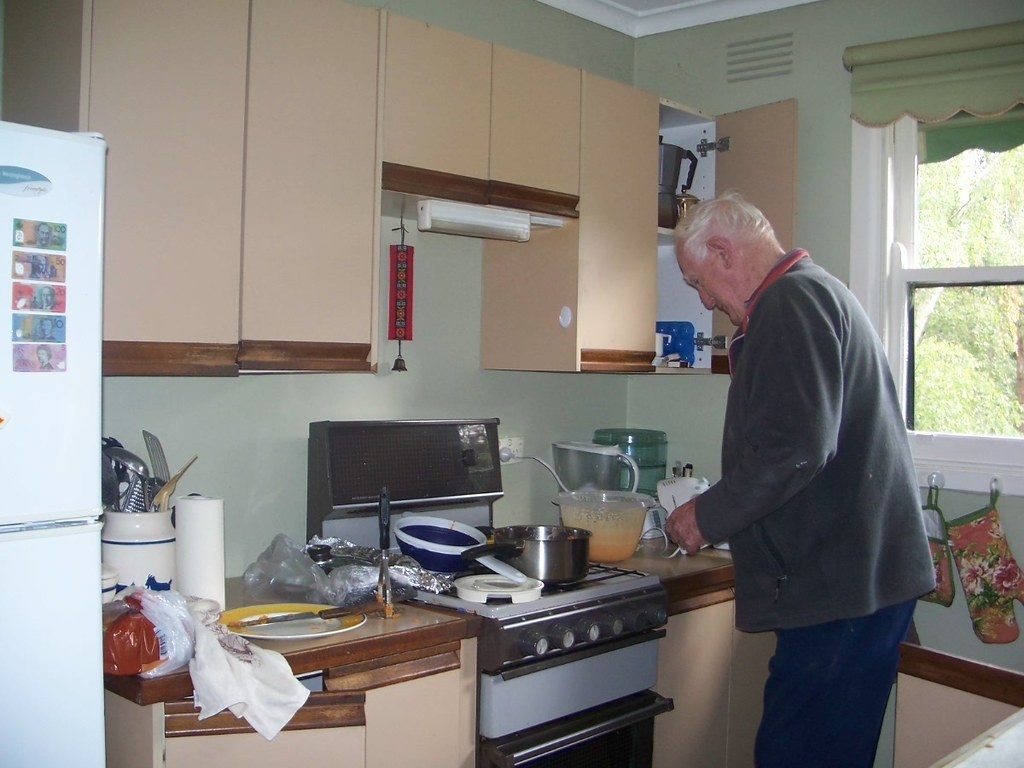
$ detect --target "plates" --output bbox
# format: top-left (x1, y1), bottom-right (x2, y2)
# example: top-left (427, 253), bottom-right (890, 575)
top-left (216, 602), bottom-right (367, 642)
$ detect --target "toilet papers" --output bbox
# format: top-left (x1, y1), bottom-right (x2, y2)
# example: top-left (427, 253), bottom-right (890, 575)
top-left (173, 492), bottom-right (227, 617)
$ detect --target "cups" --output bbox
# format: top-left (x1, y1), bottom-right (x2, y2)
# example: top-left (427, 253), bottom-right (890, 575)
top-left (675, 189), bottom-right (700, 224)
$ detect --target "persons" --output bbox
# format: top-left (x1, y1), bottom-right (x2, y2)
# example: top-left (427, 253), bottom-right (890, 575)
top-left (662, 191), bottom-right (938, 768)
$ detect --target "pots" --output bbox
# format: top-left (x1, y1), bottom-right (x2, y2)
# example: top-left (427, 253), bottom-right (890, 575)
top-left (460, 525), bottom-right (593, 584)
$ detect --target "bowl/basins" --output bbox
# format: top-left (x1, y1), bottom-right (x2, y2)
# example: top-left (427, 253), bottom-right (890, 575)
top-left (548, 486), bottom-right (655, 559)
top-left (393, 516), bottom-right (488, 572)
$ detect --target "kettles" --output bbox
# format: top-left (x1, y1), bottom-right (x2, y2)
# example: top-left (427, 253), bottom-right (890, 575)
top-left (553, 440), bottom-right (640, 492)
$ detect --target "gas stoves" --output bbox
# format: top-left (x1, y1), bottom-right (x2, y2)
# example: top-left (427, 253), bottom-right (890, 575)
top-left (393, 542), bottom-right (671, 674)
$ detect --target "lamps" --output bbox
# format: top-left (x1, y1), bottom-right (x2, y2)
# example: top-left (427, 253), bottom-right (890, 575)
top-left (415, 198), bottom-right (532, 245)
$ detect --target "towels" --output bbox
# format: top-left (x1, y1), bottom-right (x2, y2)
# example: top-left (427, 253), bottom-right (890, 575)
top-left (189, 605), bottom-right (313, 746)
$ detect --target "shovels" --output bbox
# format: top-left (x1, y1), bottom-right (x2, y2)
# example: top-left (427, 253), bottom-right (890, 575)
top-left (148, 453), bottom-right (198, 513)
top-left (143, 429), bottom-right (172, 487)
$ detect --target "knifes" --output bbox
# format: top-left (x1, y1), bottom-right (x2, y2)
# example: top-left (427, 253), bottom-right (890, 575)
top-left (224, 601), bottom-right (385, 627)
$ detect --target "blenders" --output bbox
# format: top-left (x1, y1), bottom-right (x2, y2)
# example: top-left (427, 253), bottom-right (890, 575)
top-left (657, 135), bottom-right (698, 228)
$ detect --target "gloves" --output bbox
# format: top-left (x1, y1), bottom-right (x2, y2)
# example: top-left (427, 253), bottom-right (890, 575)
top-left (946, 504), bottom-right (1024, 644)
top-left (917, 485), bottom-right (955, 606)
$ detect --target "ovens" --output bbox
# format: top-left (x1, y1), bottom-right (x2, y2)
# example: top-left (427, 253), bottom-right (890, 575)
top-left (481, 626), bottom-right (677, 767)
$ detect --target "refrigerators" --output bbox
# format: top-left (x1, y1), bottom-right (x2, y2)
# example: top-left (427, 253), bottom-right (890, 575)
top-left (0, 117), bottom-right (109, 768)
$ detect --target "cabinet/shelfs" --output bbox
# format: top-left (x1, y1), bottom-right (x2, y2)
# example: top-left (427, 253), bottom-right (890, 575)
top-left (2, 0), bottom-right (803, 373)
top-left (591, 536), bottom-right (781, 768)
top-left (105, 604), bottom-right (481, 767)
